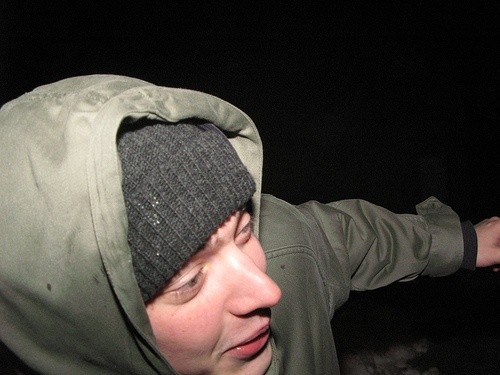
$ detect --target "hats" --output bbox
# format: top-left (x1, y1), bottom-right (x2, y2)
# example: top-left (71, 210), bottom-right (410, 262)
top-left (115, 116), bottom-right (257, 305)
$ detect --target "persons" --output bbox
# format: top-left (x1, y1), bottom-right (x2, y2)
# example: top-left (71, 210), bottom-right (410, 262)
top-left (0, 70), bottom-right (500, 375)
